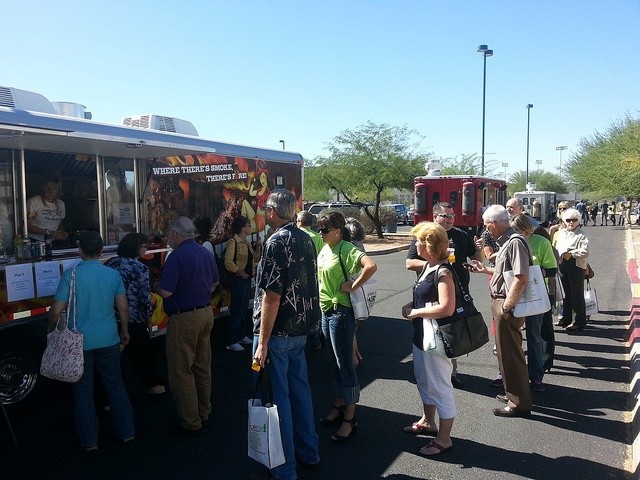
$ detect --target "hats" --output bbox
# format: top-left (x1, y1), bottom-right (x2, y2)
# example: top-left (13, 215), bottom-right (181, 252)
top-left (254, 169), bottom-right (269, 180)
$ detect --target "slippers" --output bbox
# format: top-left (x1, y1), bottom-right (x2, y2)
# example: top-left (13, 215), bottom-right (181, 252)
top-left (403, 422), bottom-right (438, 436)
top-left (419, 438), bottom-right (454, 456)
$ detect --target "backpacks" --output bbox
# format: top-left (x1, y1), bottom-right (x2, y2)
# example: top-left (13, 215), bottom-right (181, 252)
top-left (218, 237), bottom-right (237, 289)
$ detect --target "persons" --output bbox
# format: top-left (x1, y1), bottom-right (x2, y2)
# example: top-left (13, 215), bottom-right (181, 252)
top-left (143, 215), bottom-right (219, 436)
top-left (582, 209), bottom-right (589, 226)
top-left (506, 198), bottom-right (539, 234)
top-left (549, 216), bottom-right (572, 320)
top-left (617, 203), bottom-right (625, 226)
top-left (480, 229), bottom-right (498, 357)
top-left (161, 216), bottom-right (217, 264)
top-left (101, 232), bottom-right (155, 412)
top-left (404, 198), bottom-right (476, 388)
top-left (509, 212), bottom-right (556, 391)
top-left (551, 208), bottom-right (590, 336)
top-left (610, 201), bottom-right (616, 226)
top-left (344, 217), bottom-right (366, 252)
top-left (576, 200), bottom-right (585, 226)
top-left (600, 200), bottom-right (608, 226)
top-left (479, 202), bottom-right (535, 419)
top-left (44, 231), bottom-right (138, 454)
top-left (21, 173), bottom-right (68, 254)
top-left (295, 211), bottom-right (323, 351)
top-left (551, 201), bottom-right (569, 226)
top-left (626, 198), bottom-right (632, 224)
top-left (248, 187), bottom-right (323, 480)
top-left (401, 221), bottom-right (473, 459)
top-left (591, 202), bottom-right (598, 225)
top-left (221, 216), bottom-right (255, 352)
top-left (311, 213), bottom-right (377, 444)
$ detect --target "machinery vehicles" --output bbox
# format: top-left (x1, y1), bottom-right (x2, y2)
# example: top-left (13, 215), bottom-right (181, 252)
top-left (413, 168), bottom-right (506, 242)
top-left (515, 182), bottom-right (556, 224)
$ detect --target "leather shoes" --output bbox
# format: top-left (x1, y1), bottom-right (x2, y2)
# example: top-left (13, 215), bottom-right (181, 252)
top-left (493, 406), bottom-right (531, 418)
top-left (496, 394), bottom-right (509, 403)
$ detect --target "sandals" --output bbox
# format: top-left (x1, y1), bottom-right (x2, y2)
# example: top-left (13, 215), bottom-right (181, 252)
top-left (319, 407), bottom-right (345, 427)
top-left (329, 418), bottom-right (358, 442)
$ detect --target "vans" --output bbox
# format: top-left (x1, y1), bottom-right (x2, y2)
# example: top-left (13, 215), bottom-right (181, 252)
top-left (379, 204), bottom-right (408, 224)
top-left (308, 202), bottom-right (350, 215)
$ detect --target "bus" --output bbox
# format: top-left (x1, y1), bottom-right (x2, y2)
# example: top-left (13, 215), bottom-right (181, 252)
top-left (0, 106), bottom-right (305, 406)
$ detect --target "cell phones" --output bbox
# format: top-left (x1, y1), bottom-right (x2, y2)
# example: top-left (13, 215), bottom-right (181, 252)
top-left (461, 263), bottom-right (477, 273)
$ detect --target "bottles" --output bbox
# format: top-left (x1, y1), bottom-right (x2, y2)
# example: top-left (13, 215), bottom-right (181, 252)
top-left (22, 234), bottom-right (31, 261)
top-left (46, 240), bottom-right (52, 257)
top-left (14, 234), bottom-right (22, 261)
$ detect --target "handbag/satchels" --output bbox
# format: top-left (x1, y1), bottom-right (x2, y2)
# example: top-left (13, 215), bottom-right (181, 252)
top-left (339, 241), bottom-right (378, 321)
top-left (502, 236), bottom-right (552, 318)
top-left (584, 278), bottom-right (599, 316)
top-left (40, 267), bottom-right (84, 383)
top-left (555, 268), bottom-right (566, 302)
top-left (434, 263), bottom-right (489, 359)
top-left (248, 362), bottom-right (286, 470)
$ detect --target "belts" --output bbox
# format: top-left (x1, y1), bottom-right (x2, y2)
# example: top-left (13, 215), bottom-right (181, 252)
top-left (176, 302), bottom-right (212, 314)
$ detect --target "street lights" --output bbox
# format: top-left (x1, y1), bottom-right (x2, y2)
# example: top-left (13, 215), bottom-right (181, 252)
top-left (525, 104), bottom-right (533, 183)
top-left (477, 44), bottom-right (494, 176)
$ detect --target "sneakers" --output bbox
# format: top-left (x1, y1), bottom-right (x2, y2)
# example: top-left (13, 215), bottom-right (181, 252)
top-left (490, 376), bottom-right (503, 387)
top-left (226, 343), bottom-right (245, 352)
top-left (238, 336), bottom-right (253, 345)
top-left (531, 377), bottom-right (545, 391)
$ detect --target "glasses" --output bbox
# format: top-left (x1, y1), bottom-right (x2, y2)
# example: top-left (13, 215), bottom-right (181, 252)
top-left (318, 227), bottom-right (335, 235)
top-left (139, 246), bottom-right (148, 248)
top-left (437, 213), bottom-right (455, 218)
top-left (559, 208), bottom-right (567, 210)
top-left (566, 219), bottom-right (578, 222)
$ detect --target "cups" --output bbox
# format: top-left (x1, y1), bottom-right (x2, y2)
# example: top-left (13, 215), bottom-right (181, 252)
top-left (447, 248), bottom-right (456, 263)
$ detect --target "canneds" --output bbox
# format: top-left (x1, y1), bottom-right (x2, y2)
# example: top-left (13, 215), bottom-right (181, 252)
top-left (39, 242), bottom-right (46, 256)
top-left (31, 242), bottom-right (39, 258)
